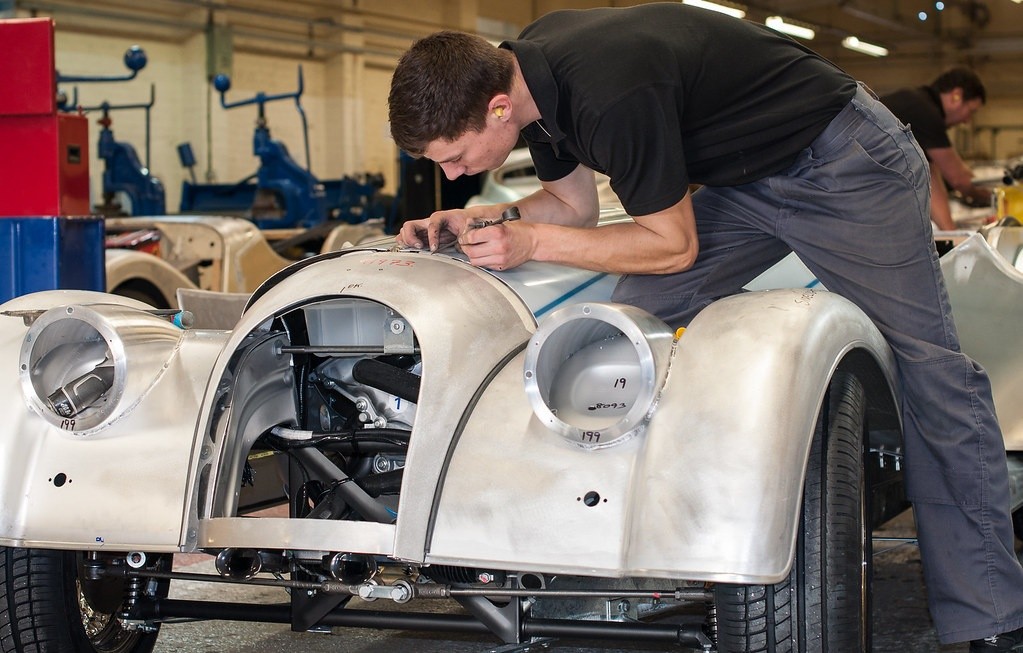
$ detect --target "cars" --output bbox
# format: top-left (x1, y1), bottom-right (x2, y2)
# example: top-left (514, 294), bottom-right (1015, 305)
top-left (0, 144), bottom-right (1023, 653)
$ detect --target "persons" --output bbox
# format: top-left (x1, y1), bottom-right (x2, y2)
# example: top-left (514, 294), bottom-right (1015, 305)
top-left (877, 68), bottom-right (994, 230)
top-left (388, 3), bottom-right (1023, 653)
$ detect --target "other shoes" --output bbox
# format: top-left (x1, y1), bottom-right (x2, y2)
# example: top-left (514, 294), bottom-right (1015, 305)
top-left (969, 636), bottom-right (1023, 653)
